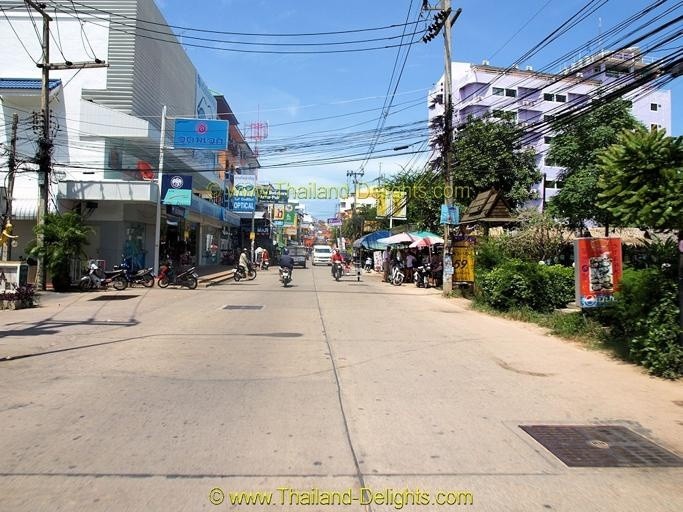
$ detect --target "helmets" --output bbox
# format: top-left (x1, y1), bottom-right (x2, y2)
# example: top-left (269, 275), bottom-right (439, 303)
top-left (243, 248), bottom-right (247, 251)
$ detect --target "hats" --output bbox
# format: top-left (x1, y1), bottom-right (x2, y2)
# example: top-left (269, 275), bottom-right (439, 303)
top-left (283, 248), bottom-right (289, 252)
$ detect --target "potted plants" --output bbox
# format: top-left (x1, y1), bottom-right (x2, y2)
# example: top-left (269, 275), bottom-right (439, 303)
top-left (25, 211), bottom-right (96, 291)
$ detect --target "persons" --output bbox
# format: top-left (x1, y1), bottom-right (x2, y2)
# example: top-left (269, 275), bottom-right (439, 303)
top-left (382, 245), bottom-right (392, 281)
top-left (279, 248), bottom-right (293, 278)
top-left (238, 248), bottom-right (249, 279)
top-left (329, 249), bottom-right (344, 276)
top-left (363, 257), bottom-right (372, 270)
top-left (259, 249), bottom-right (268, 270)
top-left (393, 248), bottom-right (442, 287)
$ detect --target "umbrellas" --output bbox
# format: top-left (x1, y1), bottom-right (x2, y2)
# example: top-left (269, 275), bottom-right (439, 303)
top-left (382, 232), bottom-right (419, 256)
top-left (408, 236), bottom-right (444, 261)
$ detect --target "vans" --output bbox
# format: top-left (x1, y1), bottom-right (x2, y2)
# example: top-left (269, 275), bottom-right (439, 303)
top-left (288, 246), bottom-right (307, 267)
top-left (312, 245), bottom-right (332, 266)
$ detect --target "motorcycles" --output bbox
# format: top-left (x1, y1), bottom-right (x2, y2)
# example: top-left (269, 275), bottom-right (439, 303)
top-left (232, 260), bottom-right (256, 281)
top-left (366, 257), bottom-right (372, 273)
top-left (413, 263), bottom-right (432, 288)
top-left (331, 260), bottom-right (342, 281)
top-left (79, 263), bottom-right (131, 291)
top-left (388, 260), bottom-right (405, 286)
top-left (260, 260), bottom-right (269, 270)
top-left (112, 263), bottom-right (155, 290)
top-left (279, 267), bottom-right (292, 288)
top-left (158, 255), bottom-right (198, 289)
